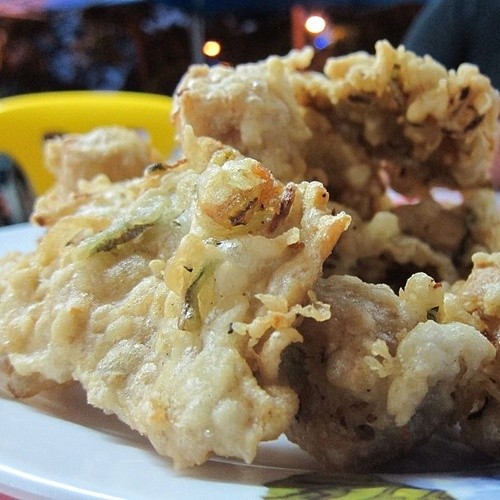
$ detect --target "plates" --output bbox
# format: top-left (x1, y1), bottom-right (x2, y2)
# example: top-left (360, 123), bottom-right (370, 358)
top-left (0, 221), bottom-right (500, 500)
